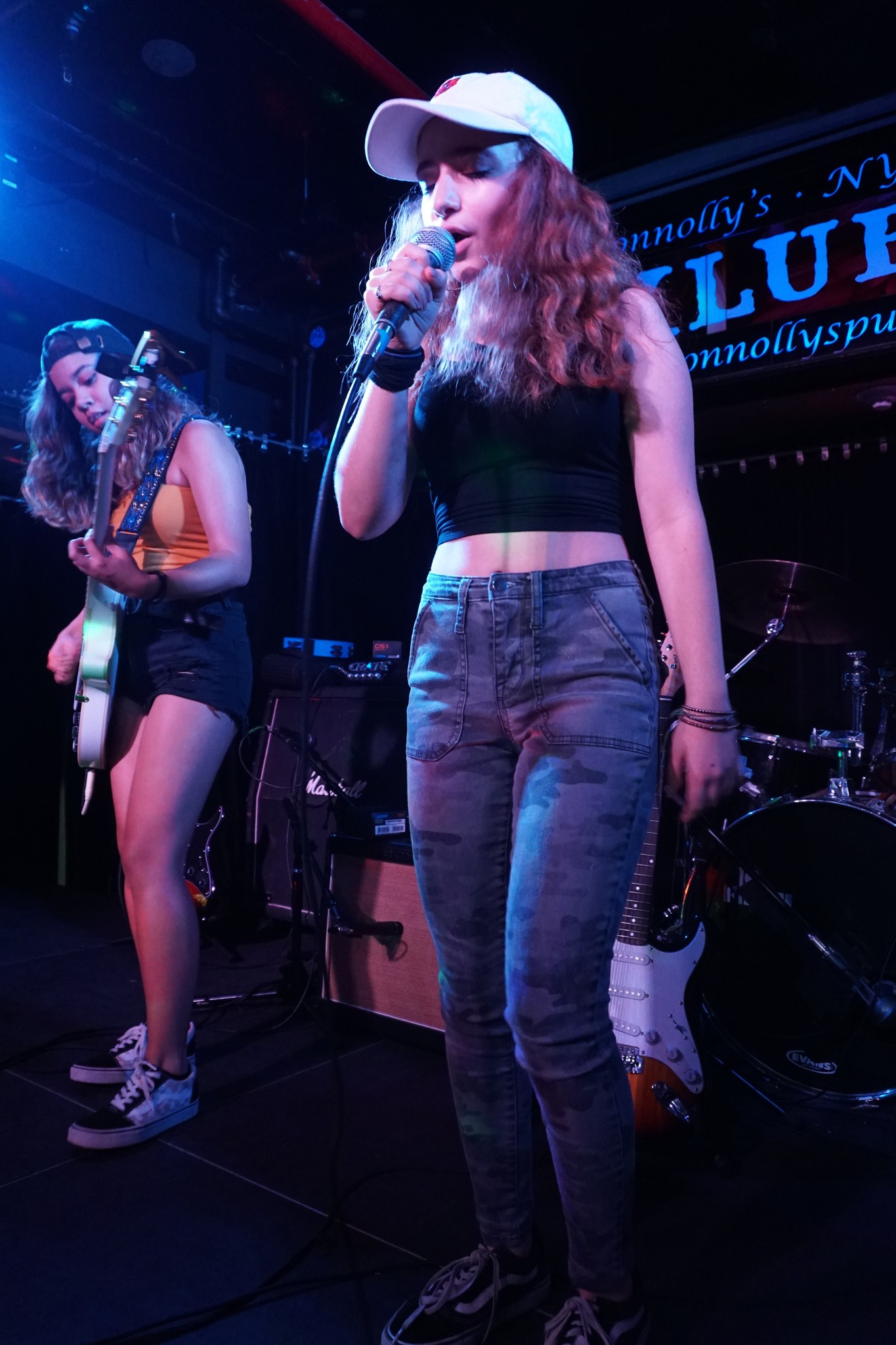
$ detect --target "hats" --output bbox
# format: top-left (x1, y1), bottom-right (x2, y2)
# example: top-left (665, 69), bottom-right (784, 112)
top-left (41, 319), bottom-right (136, 377)
top-left (365, 71), bottom-right (573, 182)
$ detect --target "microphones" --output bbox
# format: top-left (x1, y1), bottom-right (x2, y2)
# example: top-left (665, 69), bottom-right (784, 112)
top-left (335, 921), bottom-right (404, 937)
top-left (262, 725), bottom-right (315, 747)
top-left (352, 226), bottom-right (456, 384)
top-left (854, 980), bottom-right (896, 1025)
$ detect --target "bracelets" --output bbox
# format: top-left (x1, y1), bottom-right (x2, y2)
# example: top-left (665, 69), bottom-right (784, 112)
top-left (680, 705), bottom-right (739, 731)
top-left (140, 571), bottom-right (167, 605)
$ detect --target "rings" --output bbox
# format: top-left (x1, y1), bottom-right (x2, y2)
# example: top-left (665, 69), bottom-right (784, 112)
top-left (376, 285), bottom-right (382, 299)
top-left (385, 260), bottom-right (392, 272)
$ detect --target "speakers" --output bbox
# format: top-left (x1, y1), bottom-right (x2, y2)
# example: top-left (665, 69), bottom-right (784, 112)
top-left (246, 688), bottom-right (414, 929)
top-left (311, 829), bottom-right (445, 1039)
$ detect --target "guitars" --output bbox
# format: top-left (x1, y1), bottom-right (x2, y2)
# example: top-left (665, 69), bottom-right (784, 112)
top-left (69, 325), bottom-right (167, 770)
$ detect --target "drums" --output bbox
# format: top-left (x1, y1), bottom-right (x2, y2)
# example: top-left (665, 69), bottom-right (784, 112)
top-left (710, 784), bottom-right (896, 1104)
top-left (721, 729), bottom-right (836, 832)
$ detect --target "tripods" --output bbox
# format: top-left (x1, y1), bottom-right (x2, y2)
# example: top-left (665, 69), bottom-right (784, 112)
top-left (191, 799), bottom-right (350, 1041)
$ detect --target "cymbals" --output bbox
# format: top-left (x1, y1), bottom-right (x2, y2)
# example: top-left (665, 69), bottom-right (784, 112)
top-left (719, 556), bottom-right (871, 645)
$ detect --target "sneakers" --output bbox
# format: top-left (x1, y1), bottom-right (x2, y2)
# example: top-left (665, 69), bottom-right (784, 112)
top-left (70, 1021), bottom-right (196, 1084)
top-left (540, 1296), bottom-right (647, 1345)
top-left (68, 1060), bottom-right (199, 1148)
top-left (382, 1239), bottom-right (545, 1345)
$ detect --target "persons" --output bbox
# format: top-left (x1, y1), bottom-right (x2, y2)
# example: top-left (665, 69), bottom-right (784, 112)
top-left (22, 318), bottom-right (251, 1147)
top-left (336, 73), bottom-right (742, 1345)
